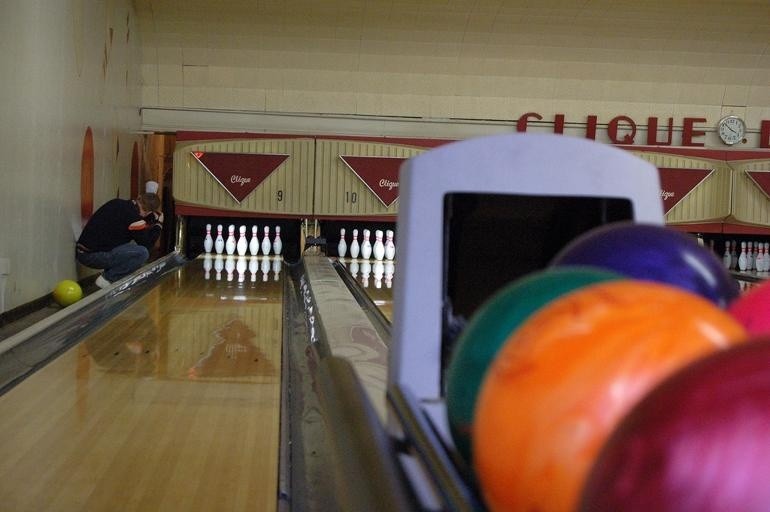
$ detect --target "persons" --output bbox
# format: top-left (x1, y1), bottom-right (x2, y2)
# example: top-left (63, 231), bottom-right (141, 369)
top-left (76, 192), bottom-right (165, 289)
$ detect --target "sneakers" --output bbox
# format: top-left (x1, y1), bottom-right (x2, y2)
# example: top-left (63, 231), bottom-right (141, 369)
top-left (95, 275), bottom-right (112, 290)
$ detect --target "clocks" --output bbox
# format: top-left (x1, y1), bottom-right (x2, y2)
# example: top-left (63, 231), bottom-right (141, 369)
top-left (718, 116), bottom-right (747, 146)
